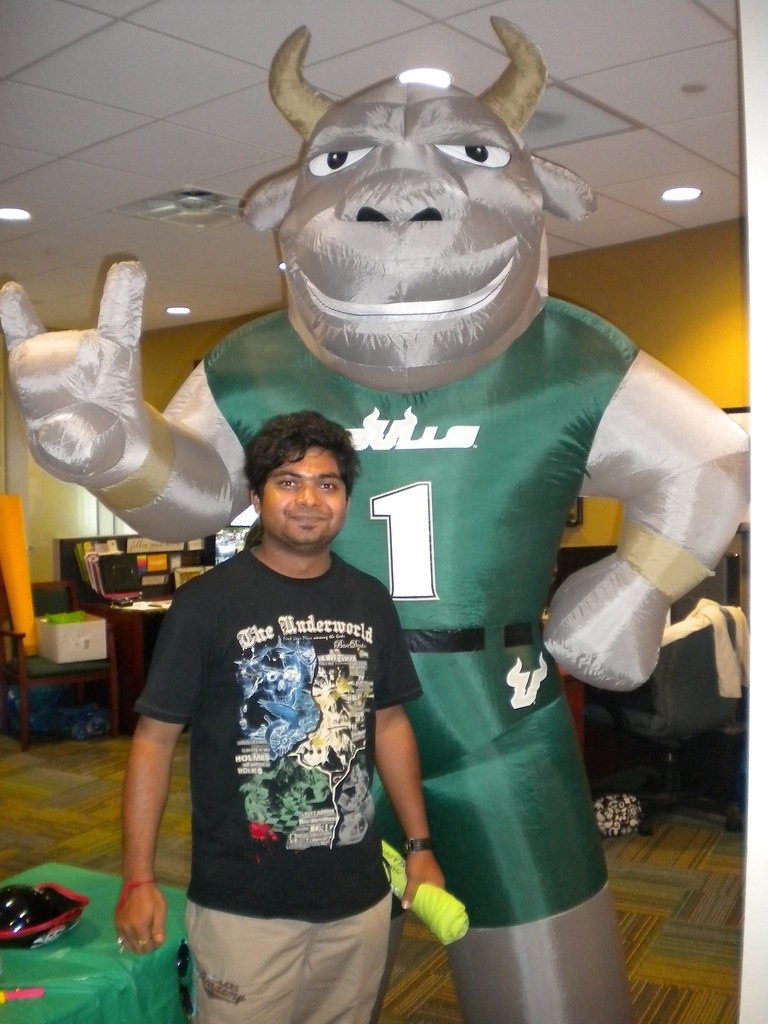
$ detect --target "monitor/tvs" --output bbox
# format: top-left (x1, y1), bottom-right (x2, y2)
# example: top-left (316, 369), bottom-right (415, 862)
top-left (205, 525), bottom-right (251, 569)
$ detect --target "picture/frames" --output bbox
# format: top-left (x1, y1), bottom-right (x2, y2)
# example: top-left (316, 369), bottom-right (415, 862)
top-left (174, 565), bottom-right (205, 593)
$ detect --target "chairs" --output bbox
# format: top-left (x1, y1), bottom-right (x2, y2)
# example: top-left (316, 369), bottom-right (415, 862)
top-left (582, 550), bottom-right (747, 838)
top-left (0, 580), bottom-right (120, 752)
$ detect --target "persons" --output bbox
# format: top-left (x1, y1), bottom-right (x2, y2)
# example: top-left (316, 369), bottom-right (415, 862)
top-left (115, 412), bottom-right (445, 1024)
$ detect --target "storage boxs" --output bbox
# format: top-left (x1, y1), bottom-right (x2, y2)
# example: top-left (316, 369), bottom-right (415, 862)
top-left (33, 610), bottom-right (108, 665)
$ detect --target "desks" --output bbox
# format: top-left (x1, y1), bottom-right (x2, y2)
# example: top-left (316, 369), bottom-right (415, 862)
top-left (0, 862), bottom-right (195, 1024)
top-left (79, 593), bottom-right (176, 738)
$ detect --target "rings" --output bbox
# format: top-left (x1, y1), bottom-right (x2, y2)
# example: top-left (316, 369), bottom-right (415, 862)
top-left (136, 940), bottom-right (147, 944)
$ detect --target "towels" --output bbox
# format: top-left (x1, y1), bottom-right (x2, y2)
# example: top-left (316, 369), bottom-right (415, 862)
top-left (663, 596), bottom-right (750, 700)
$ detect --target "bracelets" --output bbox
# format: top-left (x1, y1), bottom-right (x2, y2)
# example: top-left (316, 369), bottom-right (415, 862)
top-left (404, 837), bottom-right (432, 854)
top-left (115, 879), bottom-right (157, 917)
